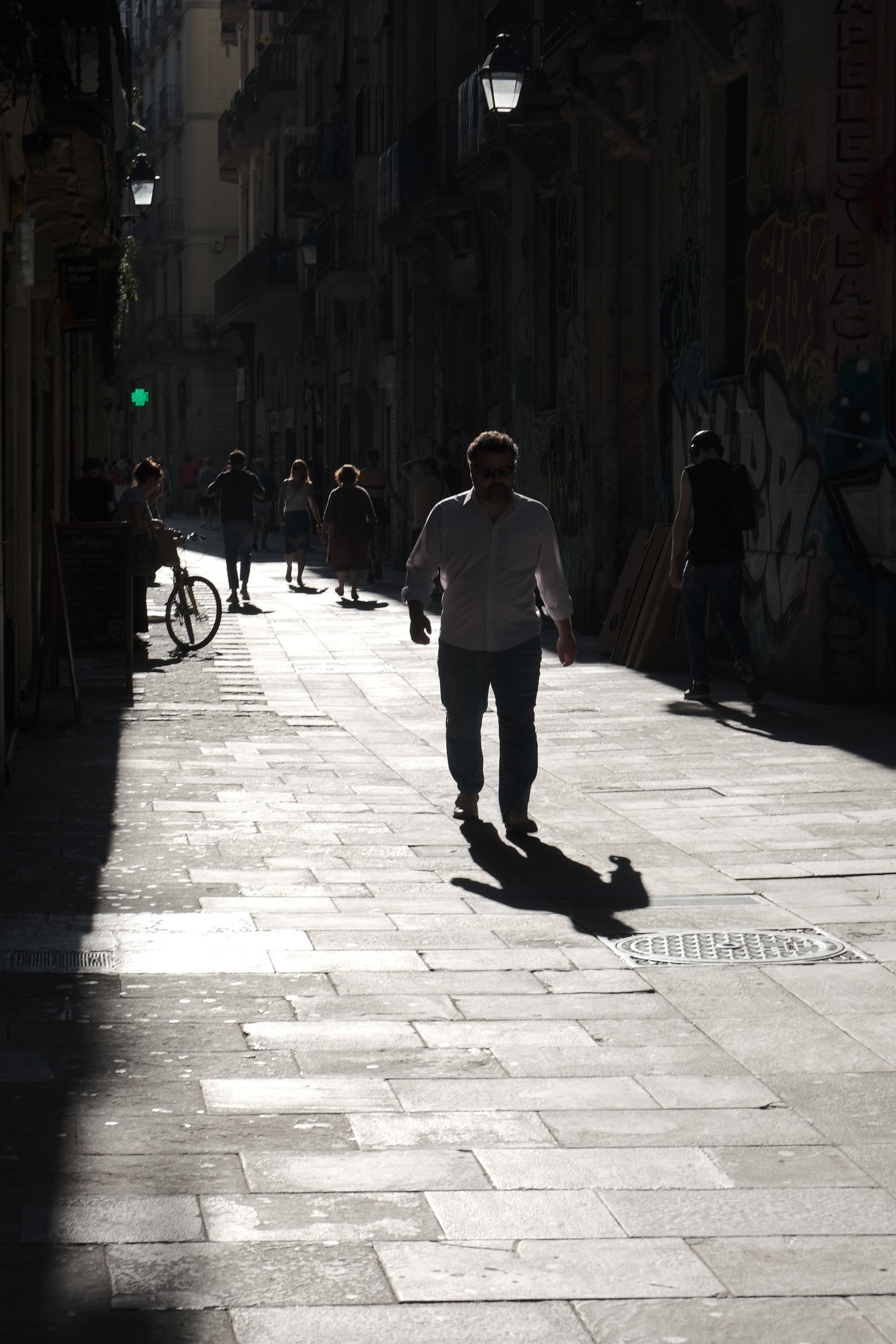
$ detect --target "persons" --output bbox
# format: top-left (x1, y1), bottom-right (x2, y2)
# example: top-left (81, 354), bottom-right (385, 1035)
top-left (206, 449), bottom-right (463, 603)
top-left (197, 457), bottom-right (219, 527)
top-left (74, 449), bottom-right (173, 650)
top-left (668, 431), bottom-right (765, 701)
top-left (401, 432), bottom-right (578, 832)
top-left (177, 452), bottom-right (199, 513)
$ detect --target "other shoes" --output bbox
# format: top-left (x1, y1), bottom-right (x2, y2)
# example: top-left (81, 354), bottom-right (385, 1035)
top-left (132, 642), bottom-right (145, 652)
top-left (226, 591), bottom-right (239, 601)
top-left (297, 573), bottom-right (303, 586)
top-left (455, 789), bottom-right (478, 819)
top-left (351, 587), bottom-right (359, 599)
top-left (286, 566), bottom-right (293, 583)
top-left (684, 685), bottom-right (710, 701)
top-left (505, 815), bottom-right (536, 836)
top-left (738, 684), bottom-right (764, 702)
top-left (335, 587), bottom-right (344, 596)
top-left (240, 584), bottom-right (250, 600)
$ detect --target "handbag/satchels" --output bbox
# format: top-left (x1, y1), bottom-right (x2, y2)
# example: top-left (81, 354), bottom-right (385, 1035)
top-left (369, 544), bottom-right (383, 584)
top-left (152, 527), bottom-right (180, 568)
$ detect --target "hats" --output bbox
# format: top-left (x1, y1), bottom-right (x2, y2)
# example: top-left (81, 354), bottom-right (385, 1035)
top-left (688, 429), bottom-right (724, 455)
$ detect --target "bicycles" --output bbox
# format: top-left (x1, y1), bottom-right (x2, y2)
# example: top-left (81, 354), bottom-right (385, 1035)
top-left (159, 524), bottom-right (222, 651)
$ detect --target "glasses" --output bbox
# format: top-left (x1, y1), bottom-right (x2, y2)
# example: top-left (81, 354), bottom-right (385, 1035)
top-left (472, 464), bottom-right (516, 477)
